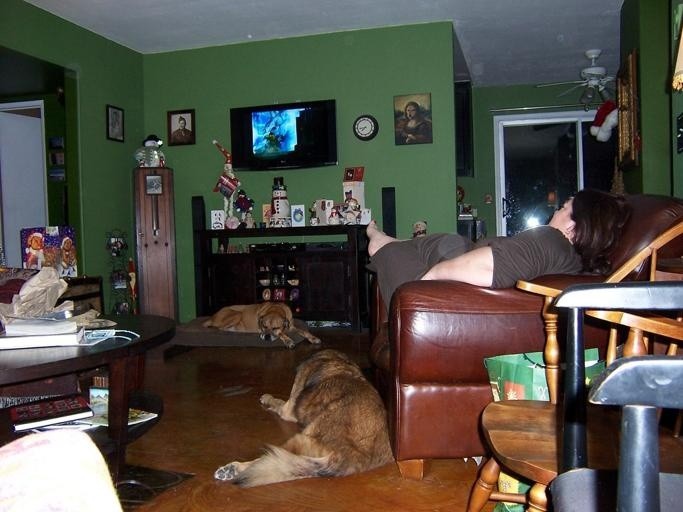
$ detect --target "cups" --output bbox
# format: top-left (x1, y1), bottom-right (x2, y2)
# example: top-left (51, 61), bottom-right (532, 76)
top-left (260, 222), bottom-right (266, 228)
top-left (250, 244), bottom-right (256, 253)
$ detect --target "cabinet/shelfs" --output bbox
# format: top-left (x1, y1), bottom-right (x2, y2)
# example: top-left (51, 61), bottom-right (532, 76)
top-left (206, 224), bottom-right (371, 333)
top-left (134, 167), bottom-right (178, 320)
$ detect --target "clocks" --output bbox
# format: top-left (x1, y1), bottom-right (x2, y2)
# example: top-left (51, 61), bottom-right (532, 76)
top-left (353, 115), bottom-right (379, 141)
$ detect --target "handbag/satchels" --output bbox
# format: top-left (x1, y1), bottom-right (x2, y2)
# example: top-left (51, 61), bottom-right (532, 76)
top-left (483, 348), bottom-right (606, 510)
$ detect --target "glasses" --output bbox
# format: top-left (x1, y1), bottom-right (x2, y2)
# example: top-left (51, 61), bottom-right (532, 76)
top-left (87, 330), bottom-right (141, 341)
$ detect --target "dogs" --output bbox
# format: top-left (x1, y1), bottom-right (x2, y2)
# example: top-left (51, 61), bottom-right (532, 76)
top-left (213, 347), bottom-right (397, 490)
top-left (200, 301), bottom-right (322, 349)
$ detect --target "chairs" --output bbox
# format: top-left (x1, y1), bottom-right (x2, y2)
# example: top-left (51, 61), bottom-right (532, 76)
top-left (467, 222), bottom-right (683, 512)
top-left (368, 196), bottom-right (682, 481)
top-left (551, 281), bottom-right (683, 512)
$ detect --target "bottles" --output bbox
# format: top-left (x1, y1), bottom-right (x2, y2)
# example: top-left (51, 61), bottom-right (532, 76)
top-left (272, 265), bottom-right (285, 285)
top-left (238, 241), bottom-right (243, 254)
top-left (139, 159), bottom-right (144, 168)
top-left (159, 156), bottom-right (164, 167)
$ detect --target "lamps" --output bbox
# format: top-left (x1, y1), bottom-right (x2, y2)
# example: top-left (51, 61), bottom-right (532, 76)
top-left (580, 77), bottom-right (616, 107)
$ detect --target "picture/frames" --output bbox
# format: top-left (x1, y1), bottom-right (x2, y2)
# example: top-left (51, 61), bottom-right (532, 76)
top-left (167, 108), bottom-right (196, 146)
top-left (106, 104), bottom-right (125, 143)
top-left (616, 48), bottom-right (638, 171)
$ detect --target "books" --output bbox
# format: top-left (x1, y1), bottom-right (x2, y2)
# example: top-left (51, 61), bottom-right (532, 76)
top-left (4, 316), bottom-right (78, 337)
top-left (9, 373), bottom-right (160, 436)
top-left (0, 327), bottom-right (85, 350)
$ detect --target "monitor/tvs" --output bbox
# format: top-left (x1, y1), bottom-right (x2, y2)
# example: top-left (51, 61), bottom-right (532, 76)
top-left (230, 99), bottom-right (338, 172)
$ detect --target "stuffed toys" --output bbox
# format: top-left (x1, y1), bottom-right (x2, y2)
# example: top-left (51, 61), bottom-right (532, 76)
top-left (589, 99), bottom-right (619, 144)
top-left (134, 134), bottom-right (167, 169)
top-left (413, 220), bottom-right (427, 239)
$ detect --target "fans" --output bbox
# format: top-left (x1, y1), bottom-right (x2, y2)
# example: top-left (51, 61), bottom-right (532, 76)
top-left (535, 49), bottom-right (617, 104)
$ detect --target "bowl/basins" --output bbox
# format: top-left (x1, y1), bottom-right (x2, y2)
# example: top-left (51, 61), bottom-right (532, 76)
top-left (259, 279), bottom-right (271, 286)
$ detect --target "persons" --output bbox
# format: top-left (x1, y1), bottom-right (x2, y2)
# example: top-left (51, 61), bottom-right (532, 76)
top-left (366, 186), bottom-right (629, 322)
top-left (396, 101), bottom-right (432, 143)
top-left (172, 116), bottom-right (193, 144)
top-left (111, 111), bottom-right (120, 129)
